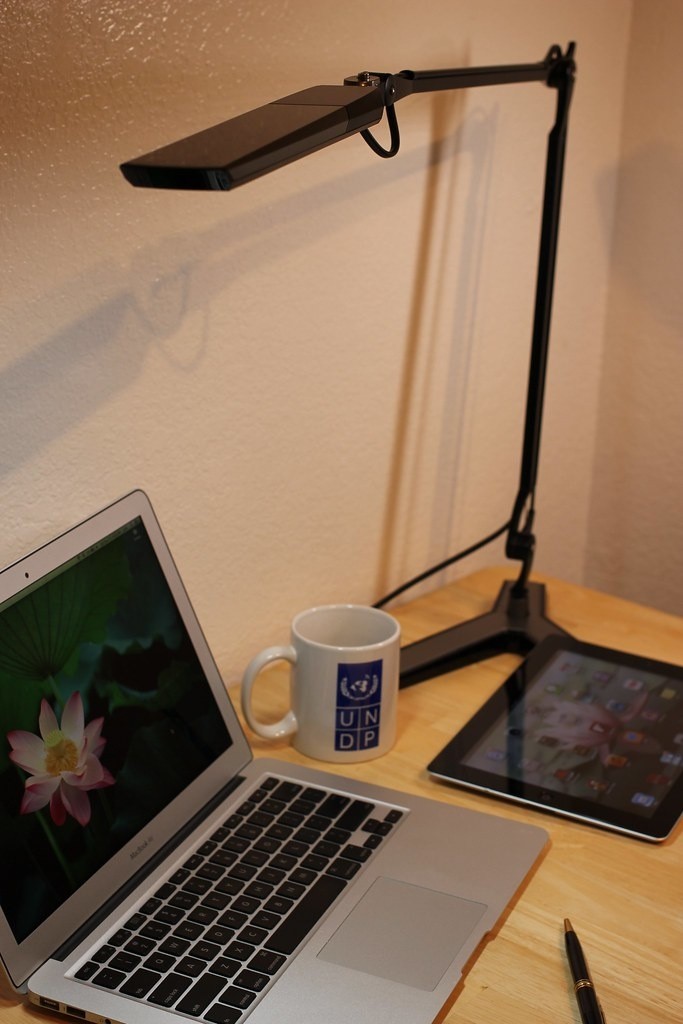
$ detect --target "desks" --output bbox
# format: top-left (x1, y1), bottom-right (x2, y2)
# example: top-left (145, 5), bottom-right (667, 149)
top-left (0, 563), bottom-right (683, 1024)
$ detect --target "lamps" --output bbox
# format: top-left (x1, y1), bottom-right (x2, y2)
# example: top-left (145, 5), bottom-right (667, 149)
top-left (116, 38), bottom-right (580, 695)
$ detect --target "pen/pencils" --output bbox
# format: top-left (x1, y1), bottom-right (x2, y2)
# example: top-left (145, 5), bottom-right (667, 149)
top-left (563, 918), bottom-right (608, 1024)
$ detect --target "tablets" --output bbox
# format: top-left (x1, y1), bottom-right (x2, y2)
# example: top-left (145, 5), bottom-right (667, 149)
top-left (425, 636), bottom-right (683, 843)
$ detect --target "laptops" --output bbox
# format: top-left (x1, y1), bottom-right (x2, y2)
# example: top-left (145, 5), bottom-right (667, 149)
top-left (0, 487), bottom-right (549, 1024)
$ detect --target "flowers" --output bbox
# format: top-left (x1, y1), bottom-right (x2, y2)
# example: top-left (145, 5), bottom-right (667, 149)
top-left (7, 692), bottom-right (115, 826)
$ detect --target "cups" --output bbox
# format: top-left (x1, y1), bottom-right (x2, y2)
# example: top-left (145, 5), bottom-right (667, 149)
top-left (240, 603), bottom-right (404, 763)
